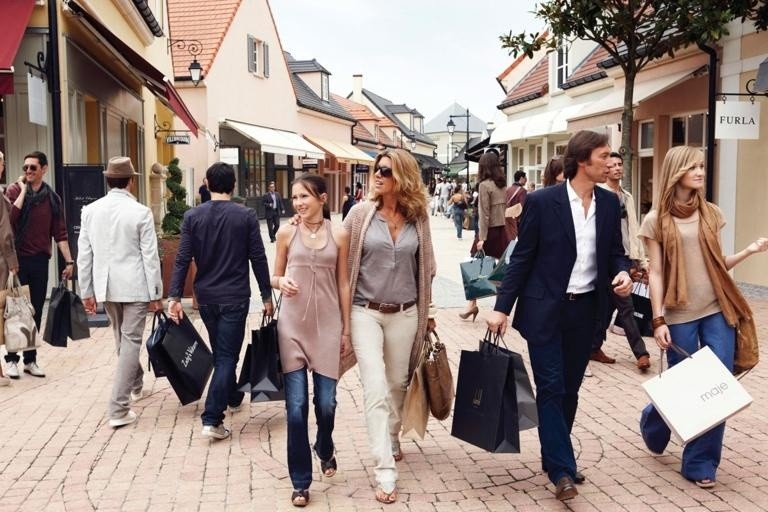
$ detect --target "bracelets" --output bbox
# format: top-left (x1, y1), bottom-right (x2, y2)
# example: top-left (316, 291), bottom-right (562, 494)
top-left (651, 315), bottom-right (667, 329)
top-left (343, 334), bottom-right (350, 336)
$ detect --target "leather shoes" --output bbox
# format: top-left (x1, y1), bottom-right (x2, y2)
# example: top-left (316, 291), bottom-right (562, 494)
top-left (638, 356), bottom-right (650, 368)
top-left (584, 369), bottom-right (593, 376)
top-left (0, 377), bottom-right (10, 385)
top-left (592, 349), bottom-right (615, 364)
top-left (6, 361), bottom-right (19, 378)
top-left (24, 363), bottom-right (46, 377)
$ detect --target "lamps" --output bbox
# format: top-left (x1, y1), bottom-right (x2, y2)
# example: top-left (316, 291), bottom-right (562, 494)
top-left (166, 34), bottom-right (205, 88)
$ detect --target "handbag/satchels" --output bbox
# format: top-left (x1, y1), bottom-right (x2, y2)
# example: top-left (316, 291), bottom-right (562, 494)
top-left (642, 343), bottom-right (753, 445)
top-left (615, 274), bottom-right (654, 336)
top-left (43, 276), bottom-right (90, 347)
top-left (401, 328), bottom-right (454, 439)
top-left (505, 203), bottom-right (523, 225)
top-left (733, 313), bottom-right (759, 376)
top-left (460, 248), bottom-right (500, 299)
top-left (451, 339), bottom-right (539, 452)
top-left (237, 308), bottom-right (286, 403)
top-left (1, 270), bottom-right (41, 352)
top-left (146, 310), bottom-right (213, 405)
top-left (487, 240), bottom-right (520, 287)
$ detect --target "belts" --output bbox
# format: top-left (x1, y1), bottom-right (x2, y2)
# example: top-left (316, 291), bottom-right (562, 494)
top-left (362, 300), bottom-right (416, 313)
top-left (564, 293), bottom-right (593, 301)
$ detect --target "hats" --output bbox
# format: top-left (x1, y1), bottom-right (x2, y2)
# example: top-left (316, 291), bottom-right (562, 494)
top-left (102, 157), bottom-right (142, 178)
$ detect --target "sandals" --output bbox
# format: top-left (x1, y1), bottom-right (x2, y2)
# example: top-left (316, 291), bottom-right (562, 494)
top-left (697, 480), bottom-right (715, 488)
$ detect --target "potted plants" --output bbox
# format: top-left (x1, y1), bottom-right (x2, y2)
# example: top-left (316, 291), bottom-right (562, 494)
top-left (157, 157), bottom-right (198, 299)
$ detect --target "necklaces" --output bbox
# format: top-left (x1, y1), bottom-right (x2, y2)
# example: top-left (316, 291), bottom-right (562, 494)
top-left (304, 220), bottom-right (325, 238)
top-left (380, 204), bottom-right (405, 230)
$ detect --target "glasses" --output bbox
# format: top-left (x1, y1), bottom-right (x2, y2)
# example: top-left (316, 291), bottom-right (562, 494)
top-left (23, 165), bottom-right (36, 171)
top-left (374, 165), bottom-right (392, 177)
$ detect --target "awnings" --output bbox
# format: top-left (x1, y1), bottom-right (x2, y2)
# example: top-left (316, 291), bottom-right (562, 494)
top-left (225, 120), bottom-right (375, 167)
top-left (414, 153), bottom-right (451, 172)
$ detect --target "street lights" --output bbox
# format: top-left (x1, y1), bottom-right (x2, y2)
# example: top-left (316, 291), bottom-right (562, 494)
top-left (446, 143), bottom-right (458, 168)
top-left (446, 108), bottom-right (470, 191)
top-left (399, 132), bottom-right (415, 152)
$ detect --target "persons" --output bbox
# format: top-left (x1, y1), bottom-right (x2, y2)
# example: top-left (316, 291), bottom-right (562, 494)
top-left (0, 149), bottom-right (74, 387)
top-left (425, 175), bottom-right (479, 238)
top-left (461, 151), bottom-right (650, 376)
top-left (261, 182), bottom-right (285, 241)
top-left (342, 182), bottom-right (363, 221)
top-left (167, 161), bottom-right (274, 440)
top-left (485, 129), bottom-right (634, 501)
top-left (198, 177), bottom-right (211, 203)
top-left (289, 147), bottom-right (437, 505)
top-left (76, 154), bottom-right (163, 427)
top-left (270, 172), bottom-right (352, 506)
top-left (636, 147), bottom-right (768, 488)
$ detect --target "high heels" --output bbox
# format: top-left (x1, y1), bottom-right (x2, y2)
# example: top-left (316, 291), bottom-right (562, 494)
top-left (459, 307), bottom-right (478, 323)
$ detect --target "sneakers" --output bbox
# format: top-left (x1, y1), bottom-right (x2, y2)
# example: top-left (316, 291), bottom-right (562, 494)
top-left (228, 405), bottom-right (242, 413)
top-left (130, 392), bottom-right (142, 400)
top-left (110, 410), bottom-right (136, 427)
top-left (203, 424), bottom-right (230, 439)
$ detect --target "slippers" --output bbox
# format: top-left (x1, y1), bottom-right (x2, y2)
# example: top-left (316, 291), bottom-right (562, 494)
top-left (291, 489), bottom-right (308, 506)
top-left (389, 434), bottom-right (402, 460)
top-left (376, 481), bottom-right (397, 503)
top-left (321, 455), bottom-right (337, 477)
